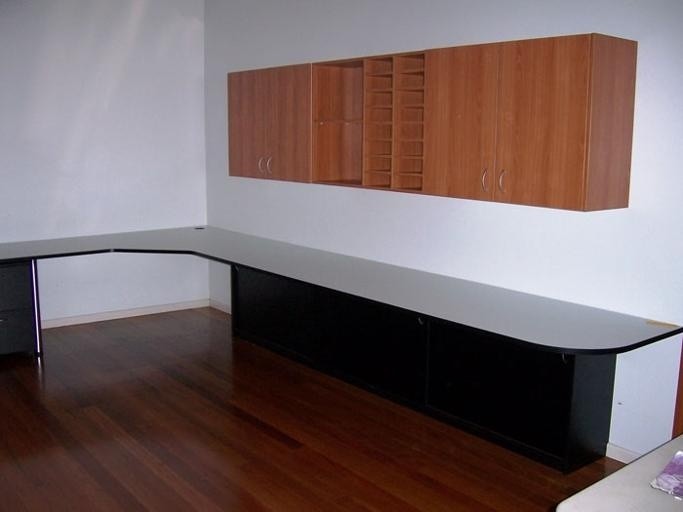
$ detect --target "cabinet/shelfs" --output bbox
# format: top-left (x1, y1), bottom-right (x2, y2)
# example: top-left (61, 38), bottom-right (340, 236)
top-left (228, 63), bottom-right (312, 185)
top-left (312, 53), bottom-right (424, 195)
top-left (230, 263), bottom-right (616, 474)
top-left (1, 257), bottom-right (36, 357)
top-left (425, 37), bottom-right (586, 211)
